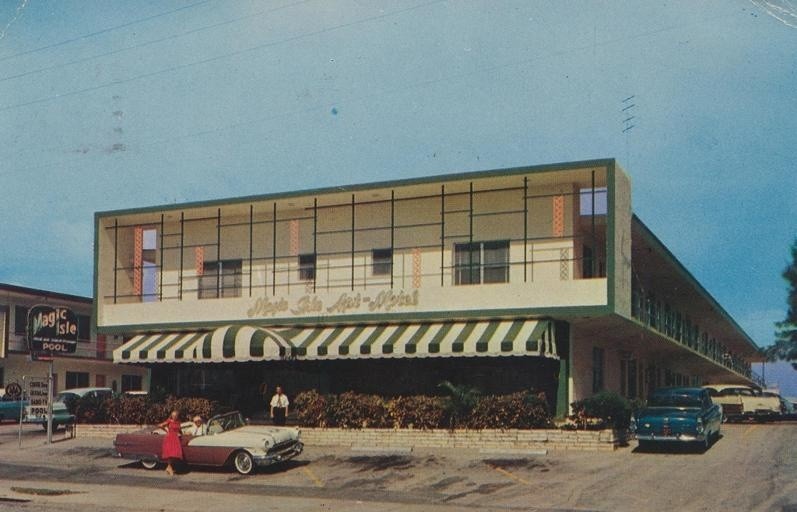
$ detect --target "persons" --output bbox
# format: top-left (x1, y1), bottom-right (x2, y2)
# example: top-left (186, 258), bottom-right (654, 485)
top-left (185, 415), bottom-right (207, 436)
top-left (269, 385), bottom-right (290, 425)
top-left (157, 410), bottom-right (184, 476)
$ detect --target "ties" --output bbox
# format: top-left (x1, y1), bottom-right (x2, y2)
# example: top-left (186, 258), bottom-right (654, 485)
top-left (278, 396), bottom-right (282, 413)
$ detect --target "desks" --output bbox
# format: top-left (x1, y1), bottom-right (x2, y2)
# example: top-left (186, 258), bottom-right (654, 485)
top-left (18, 415), bottom-right (76, 447)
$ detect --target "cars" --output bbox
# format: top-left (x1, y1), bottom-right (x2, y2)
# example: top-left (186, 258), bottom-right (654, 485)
top-left (0, 388), bottom-right (30, 425)
top-left (122, 391), bottom-right (148, 399)
top-left (22, 386), bottom-right (121, 435)
top-left (633, 384), bottom-right (726, 452)
top-left (700, 382), bottom-right (797, 423)
top-left (113, 412), bottom-right (303, 476)
